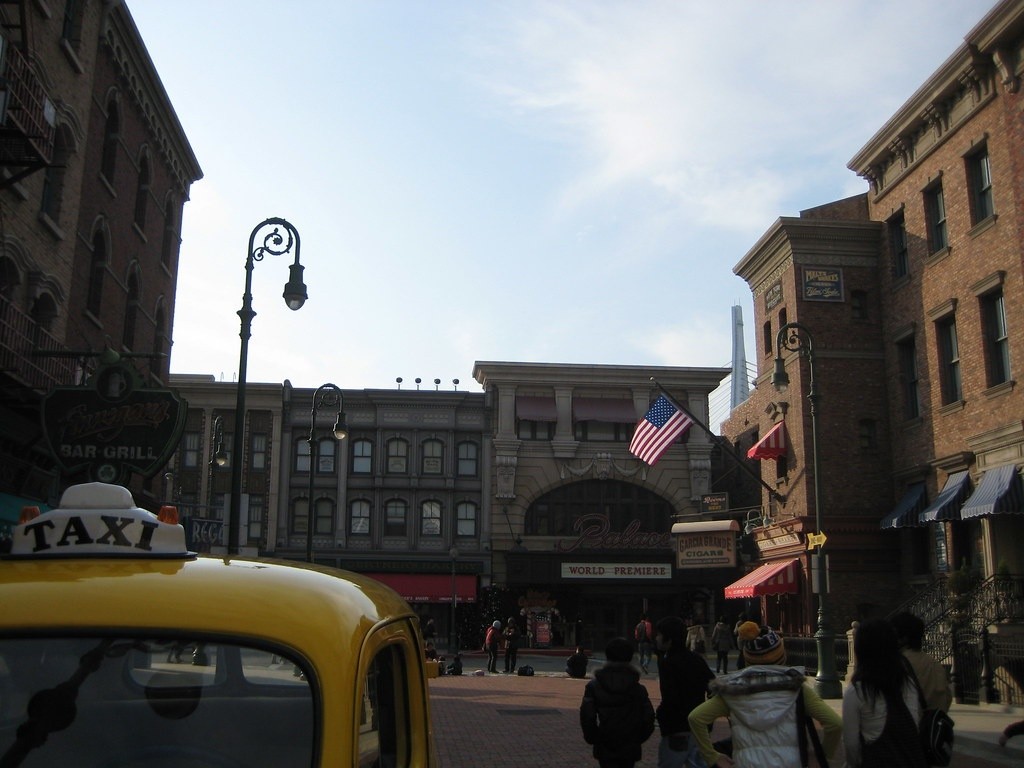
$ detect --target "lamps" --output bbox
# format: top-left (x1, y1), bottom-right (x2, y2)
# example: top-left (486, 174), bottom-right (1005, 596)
top-left (102, 346), bottom-right (120, 364)
top-left (744, 523), bottom-right (752, 534)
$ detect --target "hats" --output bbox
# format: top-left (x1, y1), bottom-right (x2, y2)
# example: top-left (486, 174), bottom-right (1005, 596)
top-left (605, 639), bottom-right (633, 661)
top-left (737, 622), bottom-right (787, 667)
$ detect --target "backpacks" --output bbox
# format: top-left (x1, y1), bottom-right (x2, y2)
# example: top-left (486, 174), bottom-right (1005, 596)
top-left (637, 622), bottom-right (646, 642)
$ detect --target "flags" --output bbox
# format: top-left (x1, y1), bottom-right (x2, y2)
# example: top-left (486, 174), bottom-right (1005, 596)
top-left (629, 395), bottom-right (694, 468)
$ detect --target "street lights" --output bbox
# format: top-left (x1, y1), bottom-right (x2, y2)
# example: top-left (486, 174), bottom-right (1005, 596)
top-left (223, 218), bottom-right (311, 554)
top-left (769, 321), bottom-right (844, 699)
top-left (449, 545), bottom-right (462, 666)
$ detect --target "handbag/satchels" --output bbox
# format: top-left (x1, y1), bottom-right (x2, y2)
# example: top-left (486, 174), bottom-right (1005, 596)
top-left (919, 708), bottom-right (955, 768)
top-left (482, 643), bottom-right (489, 654)
top-left (695, 640), bottom-right (705, 654)
top-left (518, 665), bottom-right (534, 676)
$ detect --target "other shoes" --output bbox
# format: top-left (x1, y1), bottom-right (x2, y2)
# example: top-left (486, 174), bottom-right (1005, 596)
top-left (503, 670), bottom-right (509, 672)
top-left (642, 665), bottom-right (648, 674)
top-left (491, 670), bottom-right (499, 673)
top-left (999, 728), bottom-right (1009, 746)
top-left (510, 670), bottom-right (514, 673)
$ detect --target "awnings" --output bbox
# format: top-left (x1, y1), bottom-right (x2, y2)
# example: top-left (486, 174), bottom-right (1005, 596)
top-left (516, 397), bottom-right (557, 421)
top-left (361, 572), bottom-right (479, 603)
top-left (881, 464), bottom-right (1024, 529)
top-left (747, 421), bottom-right (787, 460)
top-left (724, 560), bottom-right (800, 599)
top-left (571, 398), bottom-right (637, 424)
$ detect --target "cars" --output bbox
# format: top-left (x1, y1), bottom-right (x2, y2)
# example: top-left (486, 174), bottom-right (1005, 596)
top-left (1, 479), bottom-right (437, 768)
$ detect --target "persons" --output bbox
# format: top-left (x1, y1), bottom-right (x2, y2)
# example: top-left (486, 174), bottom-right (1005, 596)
top-left (438, 656), bottom-right (446, 672)
top-left (843, 612), bottom-right (952, 768)
top-left (502, 617), bottom-right (521, 674)
top-left (166, 641), bottom-right (206, 666)
top-left (655, 617), bottom-right (717, 768)
top-left (486, 620), bottom-right (503, 674)
top-left (579, 638), bottom-right (655, 768)
top-left (423, 617), bottom-right (438, 648)
top-left (446, 657), bottom-right (463, 675)
top-left (271, 654), bottom-right (306, 681)
top-left (688, 621), bottom-right (844, 768)
top-left (685, 612), bottom-right (748, 675)
top-left (635, 613), bottom-right (653, 674)
top-left (565, 646), bottom-right (588, 678)
top-left (424, 642), bottom-right (436, 659)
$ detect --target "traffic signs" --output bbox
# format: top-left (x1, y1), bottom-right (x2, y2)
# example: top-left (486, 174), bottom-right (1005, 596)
top-left (806, 531), bottom-right (827, 551)
